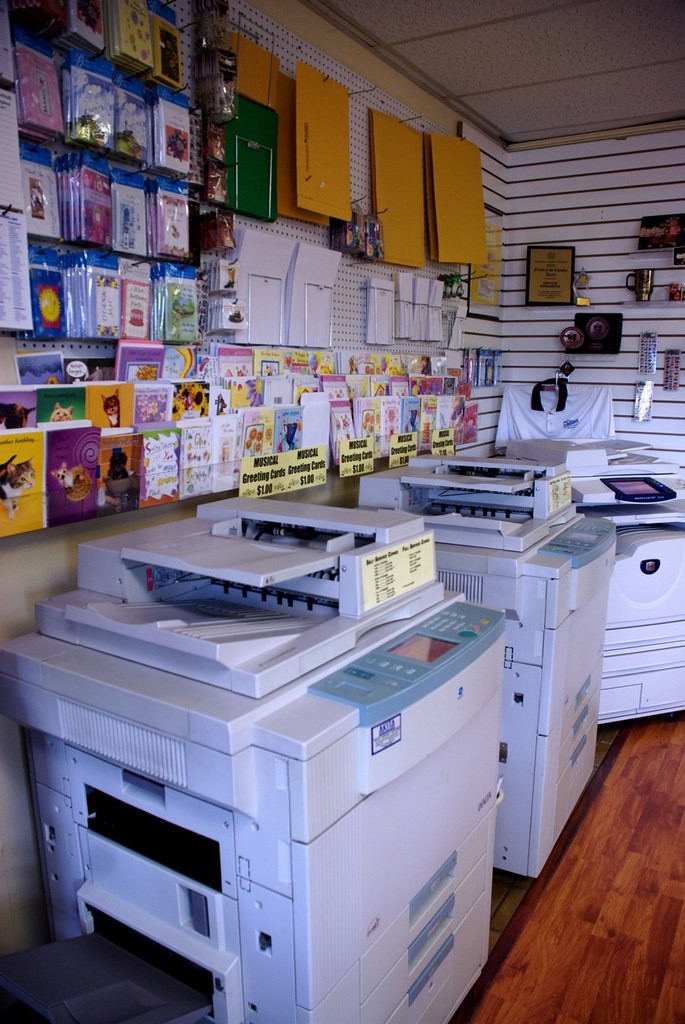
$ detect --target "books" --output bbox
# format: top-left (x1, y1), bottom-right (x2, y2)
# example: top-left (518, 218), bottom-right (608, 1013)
top-left (0, 0), bottom-right (479, 538)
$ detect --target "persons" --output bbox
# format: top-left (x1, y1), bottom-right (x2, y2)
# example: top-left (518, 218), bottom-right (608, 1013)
top-left (662, 218), bottom-right (681, 243)
top-left (103, 452), bottom-right (136, 513)
top-left (30, 181), bottom-right (42, 212)
top-left (670, 284), bottom-right (685, 301)
top-left (162, 40), bottom-right (177, 80)
